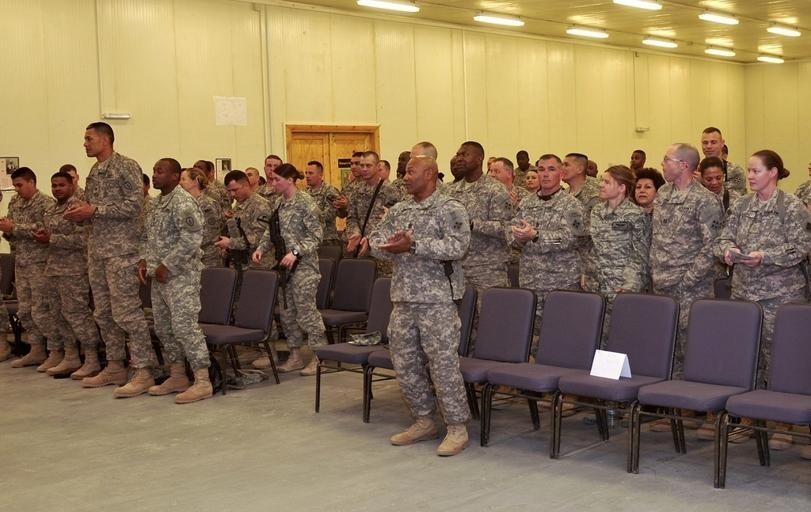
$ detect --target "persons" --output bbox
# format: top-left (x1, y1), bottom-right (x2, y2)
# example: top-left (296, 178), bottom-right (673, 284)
top-left (0, 165), bottom-right (55, 369)
top-left (336, 126), bottom-right (811, 461)
top-left (58, 121), bottom-right (345, 403)
top-left (0, 283), bottom-right (14, 364)
top-left (30, 174), bottom-right (100, 380)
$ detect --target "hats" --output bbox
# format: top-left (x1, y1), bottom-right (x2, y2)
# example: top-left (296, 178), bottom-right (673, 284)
top-left (238, 369), bottom-right (270, 385)
top-left (349, 329), bottom-right (381, 346)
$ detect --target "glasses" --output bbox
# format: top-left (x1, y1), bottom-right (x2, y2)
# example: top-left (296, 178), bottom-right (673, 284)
top-left (664, 154), bottom-right (683, 163)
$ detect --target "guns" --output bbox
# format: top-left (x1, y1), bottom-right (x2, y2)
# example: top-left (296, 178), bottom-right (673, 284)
top-left (269, 215), bottom-right (289, 311)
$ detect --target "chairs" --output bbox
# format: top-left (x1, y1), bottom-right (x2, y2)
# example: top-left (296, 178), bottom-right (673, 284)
top-left (457, 286), bottom-right (539, 448)
top-left (555, 292), bottom-right (681, 472)
top-left (0, 250), bottom-right (16, 299)
top-left (719, 300), bottom-right (811, 491)
top-left (367, 286), bottom-right (479, 424)
top-left (309, 277), bottom-right (391, 423)
top-left (633, 297), bottom-right (765, 489)
top-left (484, 290), bottom-right (607, 460)
top-left (5, 299), bottom-right (28, 364)
top-left (210, 267), bottom-right (285, 397)
top-left (196, 264), bottom-right (240, 382)
top-left (318, 259), bottom-right (377, 370)
top-left (315, 256), bottom-right (336, 311)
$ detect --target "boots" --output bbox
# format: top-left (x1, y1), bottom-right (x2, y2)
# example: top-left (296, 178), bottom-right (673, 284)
top-left (275, 349), bottom-right (305, 373)
top-left (1, 334), bottom-right (12, 362)
top-left (696, 411), bottom-right (811, 459)
top-left (299, 355), bottom-right (327, 376)
top-left (12, 342), bottom-right (81, 377)
top-left (70, 348), bottom-right (155, 397)
top-left (390, 415), bottom-right (440, 446)
top-left (477, 384), bottom-right (576, 417)
top-left (175, 367), bottom-right (213, 404)
top-left (237, 347), bottom-right (258, 363)
top-left (251, 345), bottom-right (275, 369)
top-left (436, 423), bottom-right (469, 456)
top-left (583, 401), bottom-right (696, 432)
top-left (148, 362), bottom-right (189, 395)
